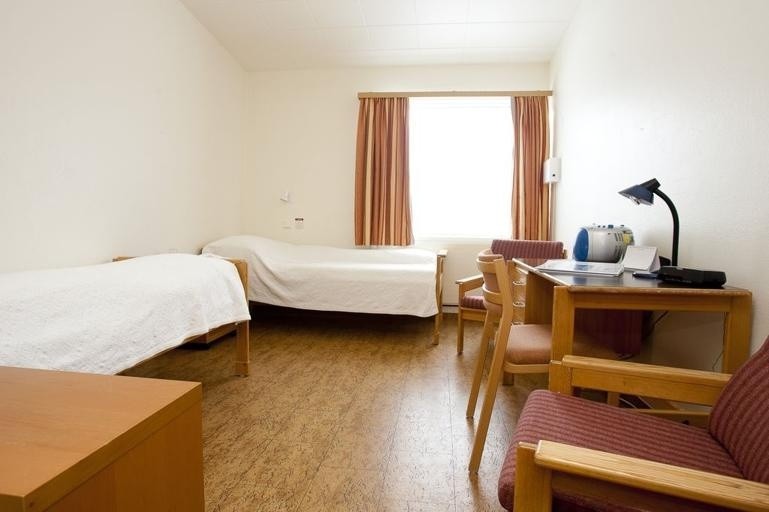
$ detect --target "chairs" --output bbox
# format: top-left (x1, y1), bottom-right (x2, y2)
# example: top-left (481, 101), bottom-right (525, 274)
top-left (498, 330), bottom-right (768, 512)
top-left (455, 238), bottom-right (622, 473)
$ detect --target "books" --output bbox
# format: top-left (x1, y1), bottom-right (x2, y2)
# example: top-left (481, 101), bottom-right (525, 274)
top-left (535, 257), bottom-right (625, 279)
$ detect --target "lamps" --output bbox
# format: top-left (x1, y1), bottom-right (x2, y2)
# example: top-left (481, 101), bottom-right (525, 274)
top-left (618, 177), bottom-right (681, 280)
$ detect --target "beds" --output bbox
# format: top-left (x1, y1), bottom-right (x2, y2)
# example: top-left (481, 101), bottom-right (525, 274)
top-left (1, 255), bottom-right (251, 375)
top-left (200, 237), bottom-right (447, 344)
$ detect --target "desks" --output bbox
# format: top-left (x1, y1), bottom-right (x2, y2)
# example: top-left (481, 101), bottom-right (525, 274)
top-left (1, 366), bottom-right (208, 512)
top-left (511, 255), bottom-right (753, 430)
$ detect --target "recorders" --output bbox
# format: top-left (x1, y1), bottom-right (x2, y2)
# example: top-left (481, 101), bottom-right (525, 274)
top-left (572, 224), bottom-right (634, 263)
